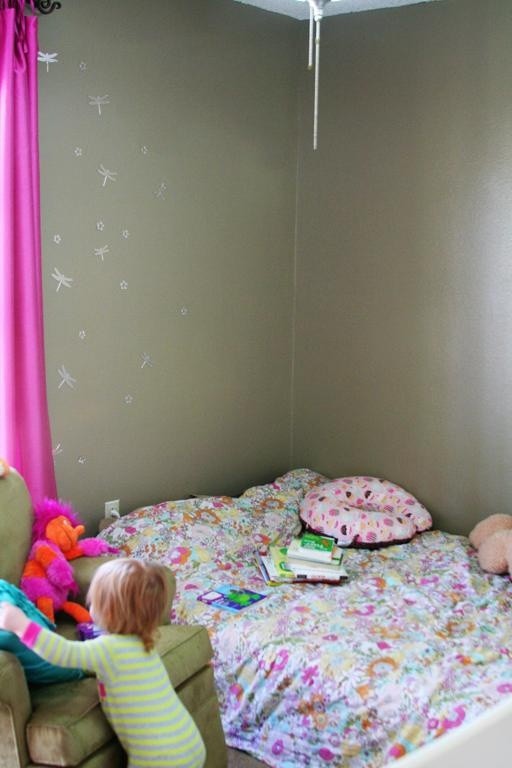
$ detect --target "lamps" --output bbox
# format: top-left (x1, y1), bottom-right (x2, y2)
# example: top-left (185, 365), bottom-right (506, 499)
top-left (306, 0), bottom-right (331, 150)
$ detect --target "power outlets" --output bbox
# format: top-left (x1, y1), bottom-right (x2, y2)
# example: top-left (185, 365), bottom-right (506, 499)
top-left (105, 499), bottom-right (119, 520)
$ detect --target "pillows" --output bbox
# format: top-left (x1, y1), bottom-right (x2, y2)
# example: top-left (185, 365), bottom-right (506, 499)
top-left (300, 475), bottom-right (433, 546)
top-left (273, 468), bottom-right (332, 497)
top-left (237, 490), bottom-right (303, 537)
top-left (1, 579), bottom-right (86, 684)
top-left (95, 498), bottom-right (257, 580)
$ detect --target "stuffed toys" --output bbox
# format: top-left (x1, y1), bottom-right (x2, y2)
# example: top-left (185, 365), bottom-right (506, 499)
top-left (467, 511), bottom-right (512, 580)
top-left (19, 495), bottom-right (121, 641)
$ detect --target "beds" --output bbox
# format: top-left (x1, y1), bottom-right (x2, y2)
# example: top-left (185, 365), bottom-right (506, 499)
top-left (92, 496), bottom-right (512, 768)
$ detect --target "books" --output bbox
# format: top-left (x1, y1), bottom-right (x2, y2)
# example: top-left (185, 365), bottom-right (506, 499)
top-left (197, 583), bottom-right (267, 614)
top-left (255, 527), bottom-right (350, 587)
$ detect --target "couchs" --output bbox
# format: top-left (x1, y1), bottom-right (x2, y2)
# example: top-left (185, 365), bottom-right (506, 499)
top-left (1, 457), bottom-right (228, 768)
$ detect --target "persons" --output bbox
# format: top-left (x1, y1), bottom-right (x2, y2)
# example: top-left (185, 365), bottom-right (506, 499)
top-left (0, 558), bottom-right (207, 768)
